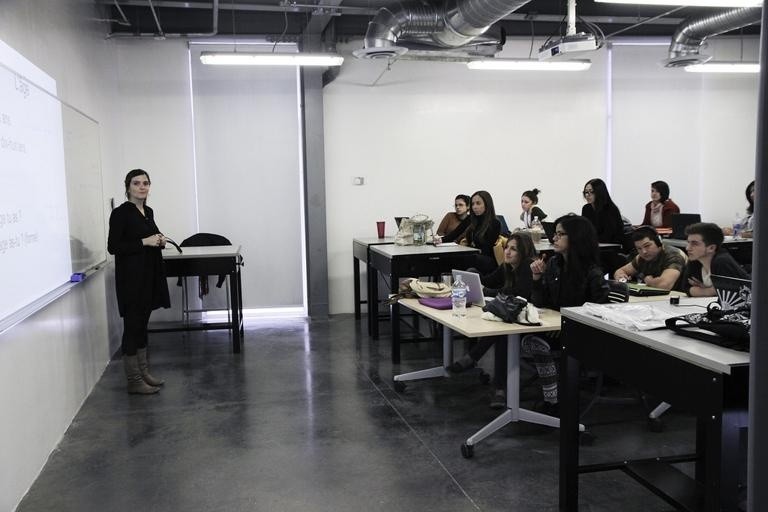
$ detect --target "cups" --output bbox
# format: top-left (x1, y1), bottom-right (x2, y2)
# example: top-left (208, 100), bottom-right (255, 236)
top-left (670, 295), bottom-right (680, 304)
top-left (376, 221), bottom-right (385, 238)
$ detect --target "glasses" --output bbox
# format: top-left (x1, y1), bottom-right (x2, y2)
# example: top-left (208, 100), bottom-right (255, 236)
top-left (553, 232), bottom-right (569, 239)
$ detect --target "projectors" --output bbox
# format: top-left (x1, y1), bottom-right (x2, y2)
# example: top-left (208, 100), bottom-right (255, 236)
top-left (538, 33), bottom-right (597, 61)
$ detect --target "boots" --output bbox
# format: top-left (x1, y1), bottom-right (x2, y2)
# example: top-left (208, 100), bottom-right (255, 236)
top-left (123, 353), bottom-right (161, 394)
top-left (137, 345), bottom-right (166, 386)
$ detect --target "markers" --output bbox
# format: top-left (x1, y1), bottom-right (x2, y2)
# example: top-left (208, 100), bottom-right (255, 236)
top-left (95, 265), bottom-right (100, 269)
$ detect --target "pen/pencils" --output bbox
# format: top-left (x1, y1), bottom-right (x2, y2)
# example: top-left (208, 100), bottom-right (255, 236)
top-left (436, 232), bottom-right (438, 240)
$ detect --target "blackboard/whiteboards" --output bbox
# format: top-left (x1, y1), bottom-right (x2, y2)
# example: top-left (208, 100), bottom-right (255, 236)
top-left (0, 39), bottom-right (107, 336)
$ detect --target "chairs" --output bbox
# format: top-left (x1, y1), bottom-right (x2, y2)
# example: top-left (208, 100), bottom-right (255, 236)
top-left (175, 232), bottom-right (243, 339)
top-left (605, 280), bottom-right (629, 303)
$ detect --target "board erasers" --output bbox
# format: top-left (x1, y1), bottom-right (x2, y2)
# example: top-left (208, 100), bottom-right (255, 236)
top-left (71, 273), bottom-right (85, 282)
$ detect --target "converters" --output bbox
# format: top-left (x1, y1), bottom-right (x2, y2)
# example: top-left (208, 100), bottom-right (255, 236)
top-left (670, 296), bottom-right (680, 305)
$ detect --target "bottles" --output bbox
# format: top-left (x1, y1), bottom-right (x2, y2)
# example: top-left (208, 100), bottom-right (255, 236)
top-left (413, 223), bottom-right (425, 246)
top-left (451, 275), bottom-right (468, 316)
top-left (530, 216), bottom-right (542, 244)
top-left (732, 212), bottom-right (744, 239)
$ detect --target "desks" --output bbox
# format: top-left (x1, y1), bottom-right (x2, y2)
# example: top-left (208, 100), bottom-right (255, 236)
top-left (662, 236), bottom-right (752, 253)
top-left (534, 238), bottom-right (622, 256)
top-left (139, 241), bottom-right (247, 356)
top-left (353, 235), bottom-right (481, 365)
top-left (623, 282), bottom-right (687, 303)
top-left (560, 297), bottom-right (752, 512)
top-left (389, 294), bottom-right (596, 460)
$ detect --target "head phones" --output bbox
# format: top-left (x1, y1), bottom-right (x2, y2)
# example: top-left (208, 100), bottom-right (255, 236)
top-left (629, 224), bottom-right (663, 248)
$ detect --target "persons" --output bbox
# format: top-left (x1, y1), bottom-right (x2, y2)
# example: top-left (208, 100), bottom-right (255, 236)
top-left (107, 169), bottom-right (171, 394)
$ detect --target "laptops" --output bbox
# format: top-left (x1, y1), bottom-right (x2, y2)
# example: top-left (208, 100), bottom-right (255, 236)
top-left (710, 273), bottom-right (752, 307)
top-left (495, 215), bottom-right (512, 234)
top-left (671, 213), bottom-right (701, 240)
top-left (394, 217), bottom-right (412, 234)
top-left (540, 221), bottom-right (556, 244)
top-left (452, 268), bottom-right (496, 308)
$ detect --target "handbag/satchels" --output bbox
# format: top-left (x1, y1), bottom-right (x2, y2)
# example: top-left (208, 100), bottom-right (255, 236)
top-left (397, 275), bottom-right (456, 300)
top-left (394, 212), bottom-right (436, 247)
top-left (416, 297), bottom-right (476, 311)
top-left (482, 290), bottom-right (544, 328)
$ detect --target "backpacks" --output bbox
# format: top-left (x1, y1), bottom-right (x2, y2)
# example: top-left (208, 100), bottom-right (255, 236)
top-left (664, 300), bottom-right (752, 354)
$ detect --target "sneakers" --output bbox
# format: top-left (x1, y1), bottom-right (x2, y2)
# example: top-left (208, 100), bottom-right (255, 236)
top-left (490, 391), bottom-right (506, 408)
top-left (445, 360), bottom-right (476, 378)
top-left (593, 381), bottom-right (644, 404)
top-left (519, 400), bottom-right (559, 437)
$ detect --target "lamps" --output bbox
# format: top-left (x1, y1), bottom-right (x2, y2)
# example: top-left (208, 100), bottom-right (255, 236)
top-left (195, 2), bottom-right (346, 71)
top-left (463, 2), bottom-right (594, 74)
top-left (685, 27), bottom-right (761, 77)
